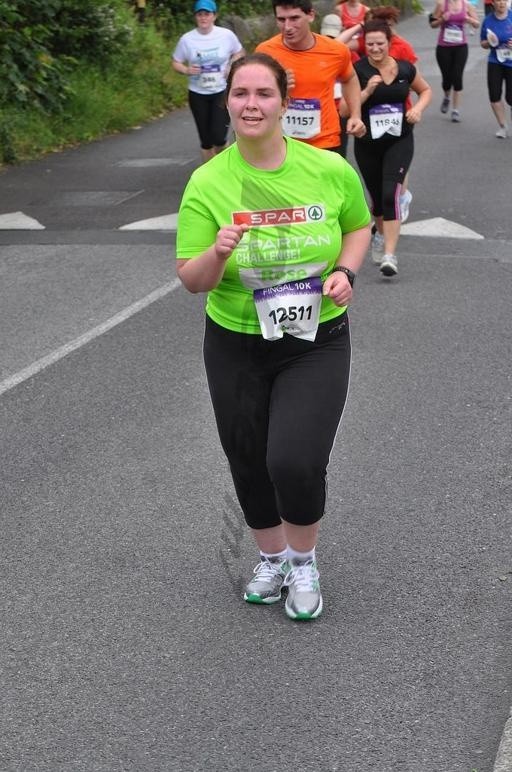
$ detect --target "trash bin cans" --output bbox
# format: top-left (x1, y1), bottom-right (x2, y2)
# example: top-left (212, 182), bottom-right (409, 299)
top-left (333, 265), bottom-right (356, 289)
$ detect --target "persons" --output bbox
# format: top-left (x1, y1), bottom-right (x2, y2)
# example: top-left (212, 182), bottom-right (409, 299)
top-left (341, 22), bottom-right (435, 276)
top-left (256, 1), bottom-right (367, 157)
top-left (171, 2), bottom-right (247, 161)
top-left (319, 0), bottom-right (512, 162)
top-left (175, 53), bottom-right (376, 623)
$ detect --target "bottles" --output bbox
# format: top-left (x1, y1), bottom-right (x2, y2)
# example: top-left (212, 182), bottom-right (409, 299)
top-left (320, 14), bottom-right (342, 38)
top-left (194, 0), bottom-right (217, 13)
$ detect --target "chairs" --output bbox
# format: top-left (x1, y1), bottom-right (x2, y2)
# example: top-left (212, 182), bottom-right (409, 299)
top-left (496, 128), bottom-right (509, 138)
top-left (371, 232), bottom-right (385, 266)
top-left (398, 189), bottom-right (412, 223)
top-left (451, 112), bottom-right (461, 122)
top-left (441, 99), bottom-right (450, 113)
top-left (244, 556), bottom-right (291, 604)
top-left (380, 254), bottom-right (398, 276)
top-left (284, 558), bottom-right (323, 620)
